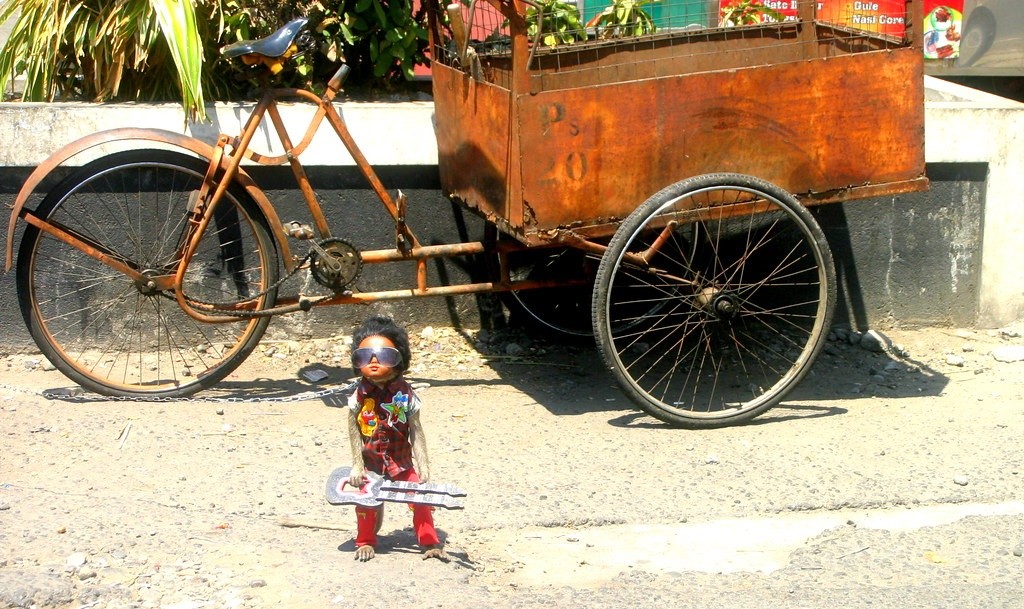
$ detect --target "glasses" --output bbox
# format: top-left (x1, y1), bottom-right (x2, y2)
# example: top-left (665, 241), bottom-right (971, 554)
top-left (351, 347), bottom-right (404, 369)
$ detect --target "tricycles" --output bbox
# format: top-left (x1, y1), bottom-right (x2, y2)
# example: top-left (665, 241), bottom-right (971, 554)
top-left (6, 0), bottom-right (930, 430)
